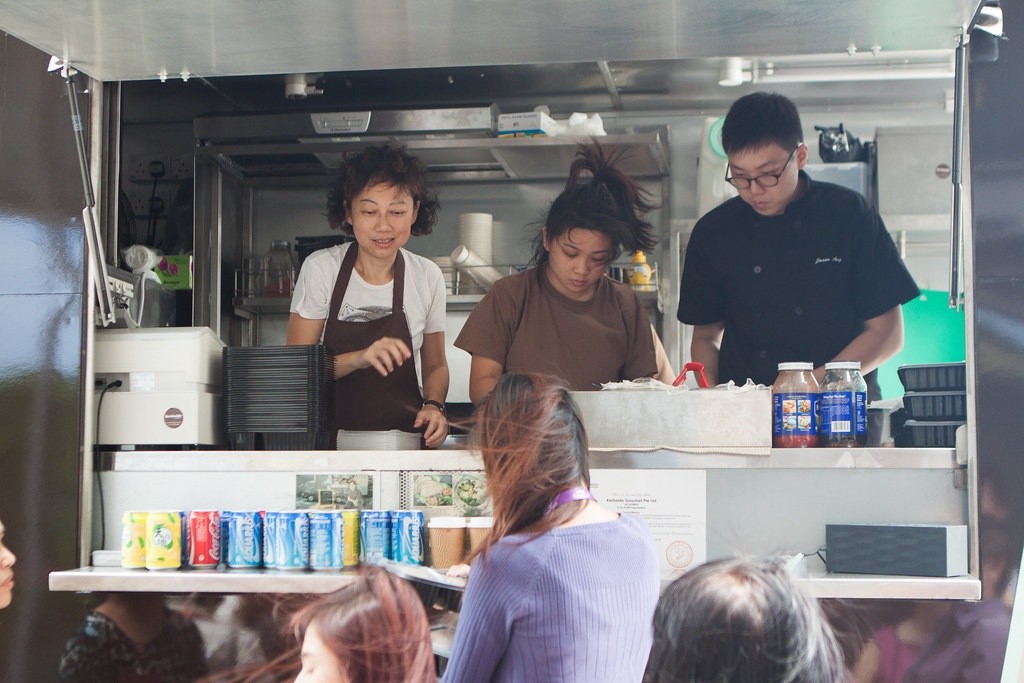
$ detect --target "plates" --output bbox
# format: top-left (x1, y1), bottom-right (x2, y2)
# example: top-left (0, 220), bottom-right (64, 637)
top-left (221, 345), bottom-right (337, 450)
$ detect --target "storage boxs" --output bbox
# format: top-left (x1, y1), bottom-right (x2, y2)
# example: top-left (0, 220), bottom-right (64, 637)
top-left (224, 345), bottom-right (337, 453)
top-left (889, 360), bottom-right (967, 447)
top-left (155, 254), bottom-right (193, 292)
top-left (803, 162), bottom-right (868, 208)
top-left (496, 110), bottom-right (559, 140)
top-left (566, 387), bottom-right (774, 449)
top-left (875, 125), bottom-right (955, 215)
top-left (97, 262), bottom-right (178, 328)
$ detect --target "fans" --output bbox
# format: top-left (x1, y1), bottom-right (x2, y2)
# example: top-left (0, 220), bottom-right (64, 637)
top-left (117, 187), bottom-right (138, 263)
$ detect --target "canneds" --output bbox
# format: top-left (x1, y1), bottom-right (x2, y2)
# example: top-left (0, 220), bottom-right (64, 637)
top-left (771, 362), bottom-right (868, 447)
top-left (120, 508), bottom-right (426, 574)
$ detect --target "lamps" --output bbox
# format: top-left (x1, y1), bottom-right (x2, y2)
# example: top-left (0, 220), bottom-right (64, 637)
top-left (718, 56), bottom-right (743, 87)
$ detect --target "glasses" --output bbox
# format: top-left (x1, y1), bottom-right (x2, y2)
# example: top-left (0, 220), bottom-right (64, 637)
top-left (726, 141), bottom-right (805, 189)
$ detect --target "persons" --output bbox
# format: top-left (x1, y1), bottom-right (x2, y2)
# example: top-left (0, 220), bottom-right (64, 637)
top-left (641, 557), bottom-right (844, 683)
top-left (855, 516), bottom-right (1017, 683)
top-left (676, 93), bottom-right (922, 389)
top-left (0, 522), bottom-right (18, 611)
top-left (293, 567), bottom-right (439, 683)
top-left (57, 591), bottom-right (299, 682)
top-left (286, 145), bottom-right (449, 449)
top-left (452, 138), bottom-right (676, 408)
top-left (438, 373), bottom-right (661, 683)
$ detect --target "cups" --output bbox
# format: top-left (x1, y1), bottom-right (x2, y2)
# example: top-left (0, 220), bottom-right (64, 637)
top-left (428, 516), bottom-right (498, 568)
top-left (427, 212), bottom-right (496, 298)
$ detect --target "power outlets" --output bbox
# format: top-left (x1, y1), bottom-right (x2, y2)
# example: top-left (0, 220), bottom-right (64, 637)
top-left (127, 187), bottom-right (170, 219)
top-left (173, 152), bottom-right (194, 181)
top-left (128, 152), bottom-right (171, 184)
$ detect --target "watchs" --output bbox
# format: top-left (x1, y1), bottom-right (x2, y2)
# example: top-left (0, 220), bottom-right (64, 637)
top-left (425, 400), bottom-right (448, 418)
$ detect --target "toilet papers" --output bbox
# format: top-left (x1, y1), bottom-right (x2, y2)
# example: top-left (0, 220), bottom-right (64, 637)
top-left (458, 212), bottom-right (494, 293)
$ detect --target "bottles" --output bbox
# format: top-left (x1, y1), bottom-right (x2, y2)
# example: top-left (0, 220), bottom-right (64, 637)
top-left (240, 239), bottom-right (297, 299)
top-left (818, 362), bottom-right (870, 447)
top-left (772, 362), bottom-right (821, 449)
